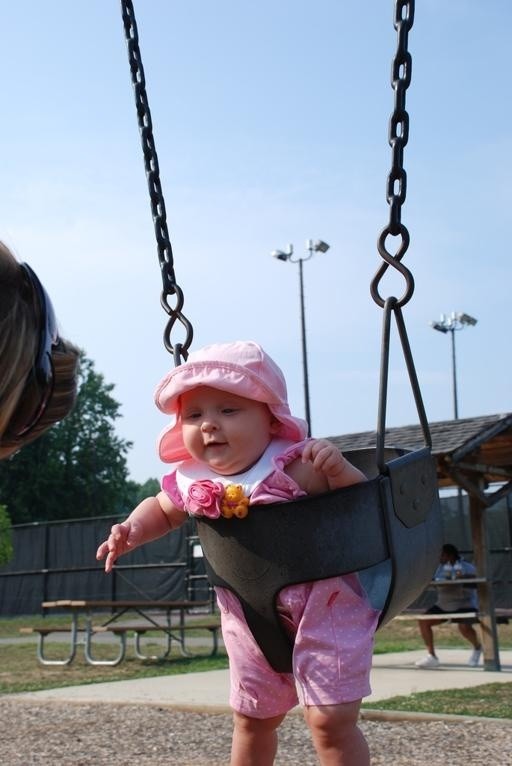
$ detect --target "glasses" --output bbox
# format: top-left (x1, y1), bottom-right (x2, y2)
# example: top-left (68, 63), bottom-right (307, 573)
top-left (2, 260), bottom-right (60, 456)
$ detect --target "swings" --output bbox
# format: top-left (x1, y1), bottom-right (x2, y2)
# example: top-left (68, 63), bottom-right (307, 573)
top-left (122, 0), bottom-right (442, 673)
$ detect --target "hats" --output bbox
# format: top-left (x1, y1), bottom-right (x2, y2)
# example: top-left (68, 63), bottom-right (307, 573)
top-left (154, 339), bottom-right (311, 464)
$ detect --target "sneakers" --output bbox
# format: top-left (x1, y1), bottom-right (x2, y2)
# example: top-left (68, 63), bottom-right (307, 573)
top-left (414, 654), bottom-right (440, 668)
top-left (468, 647), bottom-right (483, 667)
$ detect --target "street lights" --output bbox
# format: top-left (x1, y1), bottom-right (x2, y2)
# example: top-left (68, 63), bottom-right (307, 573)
top-left (427, 310), bottom-right (480, 420)
top-left (271, 236), bottom-right (329, 446)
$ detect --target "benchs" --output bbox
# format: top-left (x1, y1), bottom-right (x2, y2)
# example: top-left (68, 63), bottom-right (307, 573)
top-left (391, 605), bottom-right (512, 623)
top-left (19, 599), bottom-right (221, 667)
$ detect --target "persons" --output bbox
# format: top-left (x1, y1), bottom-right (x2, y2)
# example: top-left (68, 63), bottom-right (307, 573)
top-left (95, 340), bottom-right (391, 766)
top-left (416, 543), bottom-right (486, 667)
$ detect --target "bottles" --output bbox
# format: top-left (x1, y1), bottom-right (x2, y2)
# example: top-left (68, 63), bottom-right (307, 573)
top-left (444, 560), bottom-right (462, 579)
